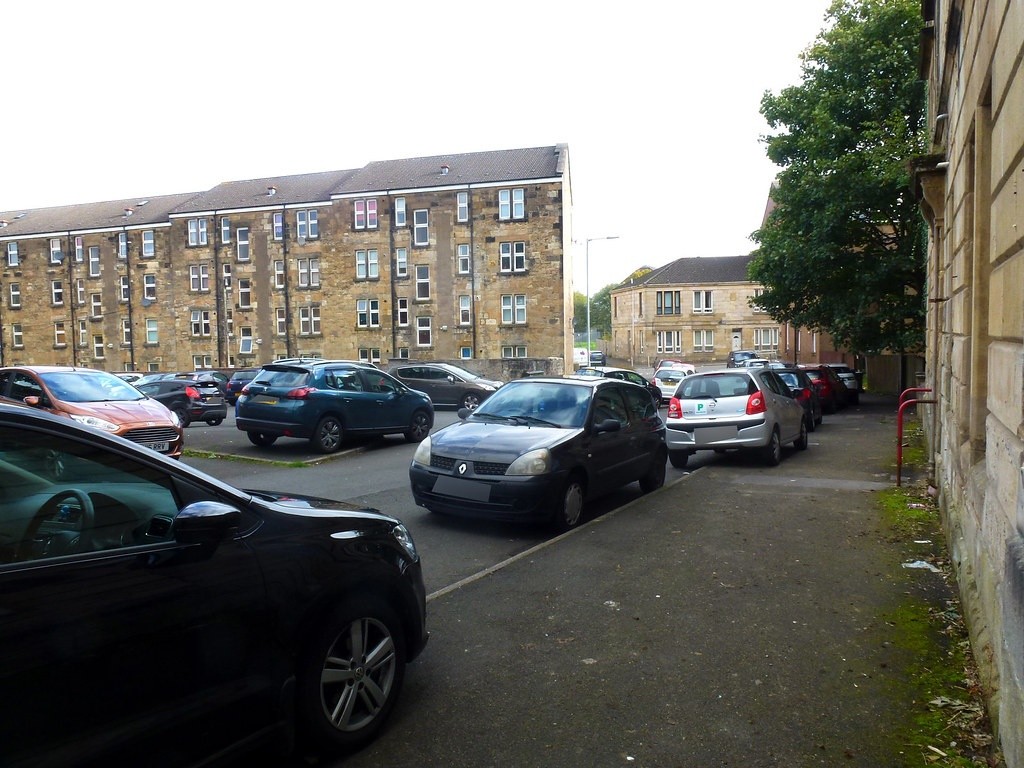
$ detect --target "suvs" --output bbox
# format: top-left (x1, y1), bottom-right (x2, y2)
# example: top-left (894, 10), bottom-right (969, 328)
top-left (235, 358), bottom-right (434, 454)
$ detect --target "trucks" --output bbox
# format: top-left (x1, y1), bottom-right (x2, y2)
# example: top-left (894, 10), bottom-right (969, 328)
top-left (573, 348), bottom-right (588, 367)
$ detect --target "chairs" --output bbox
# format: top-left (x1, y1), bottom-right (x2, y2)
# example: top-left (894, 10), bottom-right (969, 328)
top-left (338, 374), bottom-right (357, 390)
top-left (706, 381), bottom-right (720, 396)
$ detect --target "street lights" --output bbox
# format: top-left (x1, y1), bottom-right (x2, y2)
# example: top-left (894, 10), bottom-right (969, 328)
top-left (586, 236), bottom-right (619, 368)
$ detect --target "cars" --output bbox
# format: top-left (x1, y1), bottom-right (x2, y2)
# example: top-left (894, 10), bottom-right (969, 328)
top-left (224, 369), bottom-right (261, 406)
top-left (410, 376), bottom-right (669, 531)
top-left (671, 364), bottom-right (696, 376)
top-left (665, 367), bottom-right (808, 469)
top-left (575, 367), bottom-right (662, 410)
top-left (589, 351), bottom-right (606, 366)
top-left (0, 394), bottom-right (431, 768)
top-left (726, 350), bottom-right (759, 369)
top-left (786, 364), bottom-right (850, 413)
top-left (734, 368), bottom-right (823, 432)
top-left (765, 363), bottom-right (797, 369)
top-left (129, 380), bottom-right (228, 427)
top-left (0, 365), bottom-right (185, 480)
top-left (651, 367), bottom-right (687, 401)
top-left (828, 365), bottom-right (859, 404)
top-left (380, 363), bottom-right (504, 412)
top-left (654, 359), bottom-right (682, 374)
top-left (739, 359), bottom-right (769, 368)
top-left (101, 373), bottom-right (142, 386)
top-left (109, 374), bottom-right (176, 399)
top-left (174, 370), bottom-right (230, 403)
